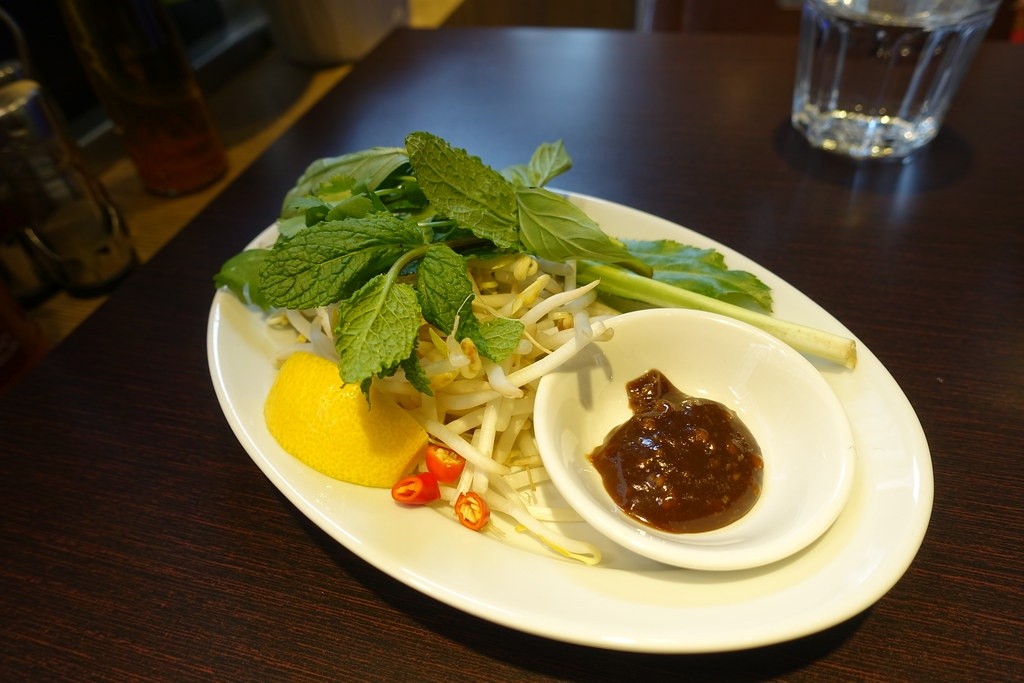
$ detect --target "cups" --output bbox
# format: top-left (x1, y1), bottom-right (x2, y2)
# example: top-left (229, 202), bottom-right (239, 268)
top-left (791, 0), bottom-right (1000, 159)
top-left (63, 0), bottom-right (227, 199)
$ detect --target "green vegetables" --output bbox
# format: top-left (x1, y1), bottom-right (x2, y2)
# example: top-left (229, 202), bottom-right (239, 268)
top-left (212, 130), bottom-right (857, 414)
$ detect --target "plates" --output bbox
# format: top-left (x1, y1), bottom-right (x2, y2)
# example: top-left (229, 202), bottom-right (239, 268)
top-left (534, 309), bottom-right (854, 570)
top-left (207, 188), bottom-right (935, 654)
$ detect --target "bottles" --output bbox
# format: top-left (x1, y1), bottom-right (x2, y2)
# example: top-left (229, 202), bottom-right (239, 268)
top-left (0, 10), bottom-right (139, 301)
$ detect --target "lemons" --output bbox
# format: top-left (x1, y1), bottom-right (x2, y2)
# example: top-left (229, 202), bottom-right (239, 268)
top-left (262, 351), bottom-right (430, 488)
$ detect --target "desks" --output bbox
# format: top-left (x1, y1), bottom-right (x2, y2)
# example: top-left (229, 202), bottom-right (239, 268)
top-left (0, 0), bottom-right (1024, 683)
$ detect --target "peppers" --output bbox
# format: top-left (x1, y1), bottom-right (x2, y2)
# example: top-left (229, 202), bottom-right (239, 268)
top-left (454, 491), bottom-right (491, 532)
top-left (391, 471), bottom-right (442, 505)
top-left (425, 445), bottom-right (465, 484)
